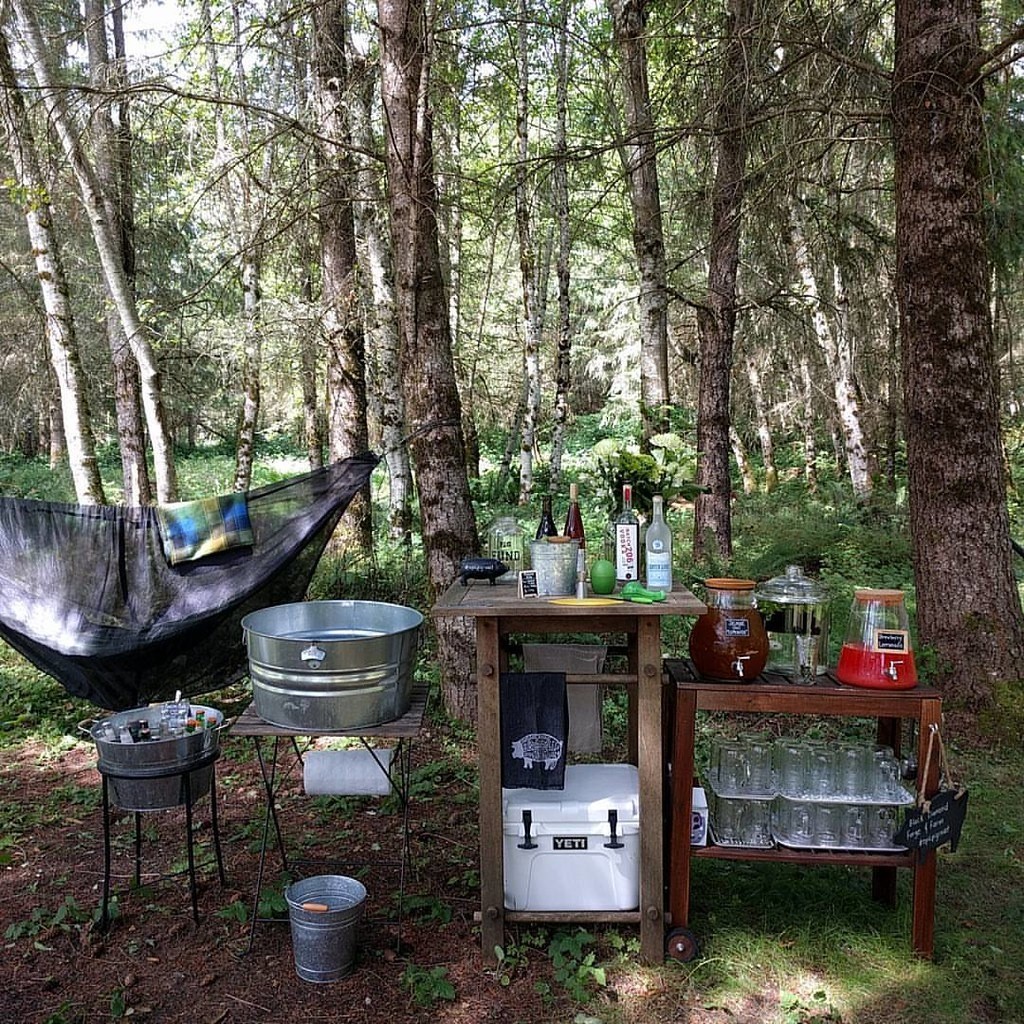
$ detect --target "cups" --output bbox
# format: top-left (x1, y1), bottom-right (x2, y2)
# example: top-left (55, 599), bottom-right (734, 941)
top-left (711, 732), bottom-right (900, 847)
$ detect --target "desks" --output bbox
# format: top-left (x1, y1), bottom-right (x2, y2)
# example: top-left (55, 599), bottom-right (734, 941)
top-left (659, 658), bottom-right (942, 963)
top-left (229, 679), bottom-right (433, 956)
top-left (431, 572), bottom-right (709, 968)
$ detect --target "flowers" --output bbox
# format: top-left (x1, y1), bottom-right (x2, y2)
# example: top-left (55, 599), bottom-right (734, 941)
top-left (587, 432), bottom-right (709, 510)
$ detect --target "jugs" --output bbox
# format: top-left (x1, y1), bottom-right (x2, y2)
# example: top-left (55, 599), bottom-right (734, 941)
top-left (838, 589), bottom-right (917, 689)
top-left (689, 577), bottom-right (769, 680)
top-left (755, 567), bottom-right (834, 674)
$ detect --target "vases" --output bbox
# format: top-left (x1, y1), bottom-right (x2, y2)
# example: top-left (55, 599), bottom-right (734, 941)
top-left (604, 501), bottom-right (674, 581)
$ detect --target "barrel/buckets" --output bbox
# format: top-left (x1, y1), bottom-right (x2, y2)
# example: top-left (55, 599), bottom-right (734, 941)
top-left (529, 543), bottom-right (578, 596)
top-left (286, 874), bottom-right (365, 983)
top-left (76, 704), bottom-right (225, 812)
top-left (242, 599), bottom-right (426, 731)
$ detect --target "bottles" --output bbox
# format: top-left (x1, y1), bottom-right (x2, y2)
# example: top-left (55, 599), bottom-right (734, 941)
top-left (564, 484), bottom-right (586, 580)
top-left (536, 496), bottom-right (557, 540)
top-left (614, 485), bottom-right (640, 588)
top-left (95, 710), bottom-right (216, 744)
top-left (647, 493), bottom-right (671, 591)
top-left (489, 516), bottom-right (525, 582)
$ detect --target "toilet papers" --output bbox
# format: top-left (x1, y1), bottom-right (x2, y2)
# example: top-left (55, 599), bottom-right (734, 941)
top-left (302, 748), bottom-right (395, 796)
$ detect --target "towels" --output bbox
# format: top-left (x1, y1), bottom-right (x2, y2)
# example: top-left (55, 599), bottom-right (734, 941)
top-left (499, 672), bottom-right (569, 791)
top-left (523, 642), bottom-right (608, 752)
top-left (153, 490), bottom-right (257, 568)
